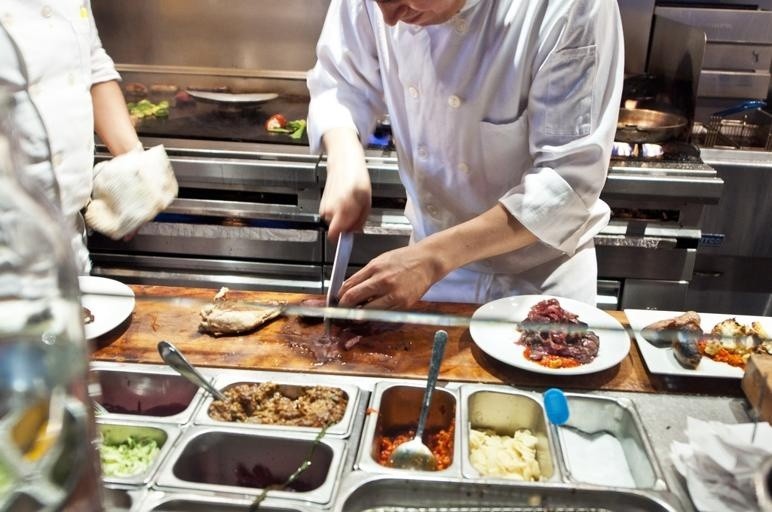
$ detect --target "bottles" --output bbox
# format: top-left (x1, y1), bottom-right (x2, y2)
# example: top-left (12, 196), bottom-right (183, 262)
top-left (0, 23), bottom-right (110, 510)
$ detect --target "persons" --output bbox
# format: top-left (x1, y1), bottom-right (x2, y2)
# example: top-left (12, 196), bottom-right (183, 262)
top-left (306, 0), bottom-right (627, 349)
top-left (0, 0), bottom-right (150, 277)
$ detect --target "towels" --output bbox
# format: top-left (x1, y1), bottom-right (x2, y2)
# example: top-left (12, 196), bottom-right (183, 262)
top-left (83, 142), bottom-right (179, 239)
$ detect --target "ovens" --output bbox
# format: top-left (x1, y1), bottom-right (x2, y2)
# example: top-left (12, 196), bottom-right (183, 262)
top-left (321, 184), bottom-right (707, 320)
top-left (82, 147), bottom-right (322, 290)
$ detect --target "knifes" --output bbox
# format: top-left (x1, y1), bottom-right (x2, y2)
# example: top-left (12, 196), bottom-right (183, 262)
top-left (322, 227), bottom-right (358, 334)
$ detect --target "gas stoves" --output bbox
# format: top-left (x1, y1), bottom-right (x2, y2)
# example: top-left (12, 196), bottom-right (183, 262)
top-left (320, 65), bottom-right (725, 204)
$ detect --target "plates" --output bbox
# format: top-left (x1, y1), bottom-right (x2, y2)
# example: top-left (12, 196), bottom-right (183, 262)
top-left (78, 274), bottom-right (138, 347)
top-left (181, 87), bottom-right (278, 110)
top-left (623, 308), bottom-right (771, 378)
top-left (470, 289), bottom-right (630, 377)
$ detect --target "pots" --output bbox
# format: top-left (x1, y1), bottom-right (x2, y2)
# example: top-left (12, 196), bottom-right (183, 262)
top-left (615, 109), bottom-right (693, 146)
